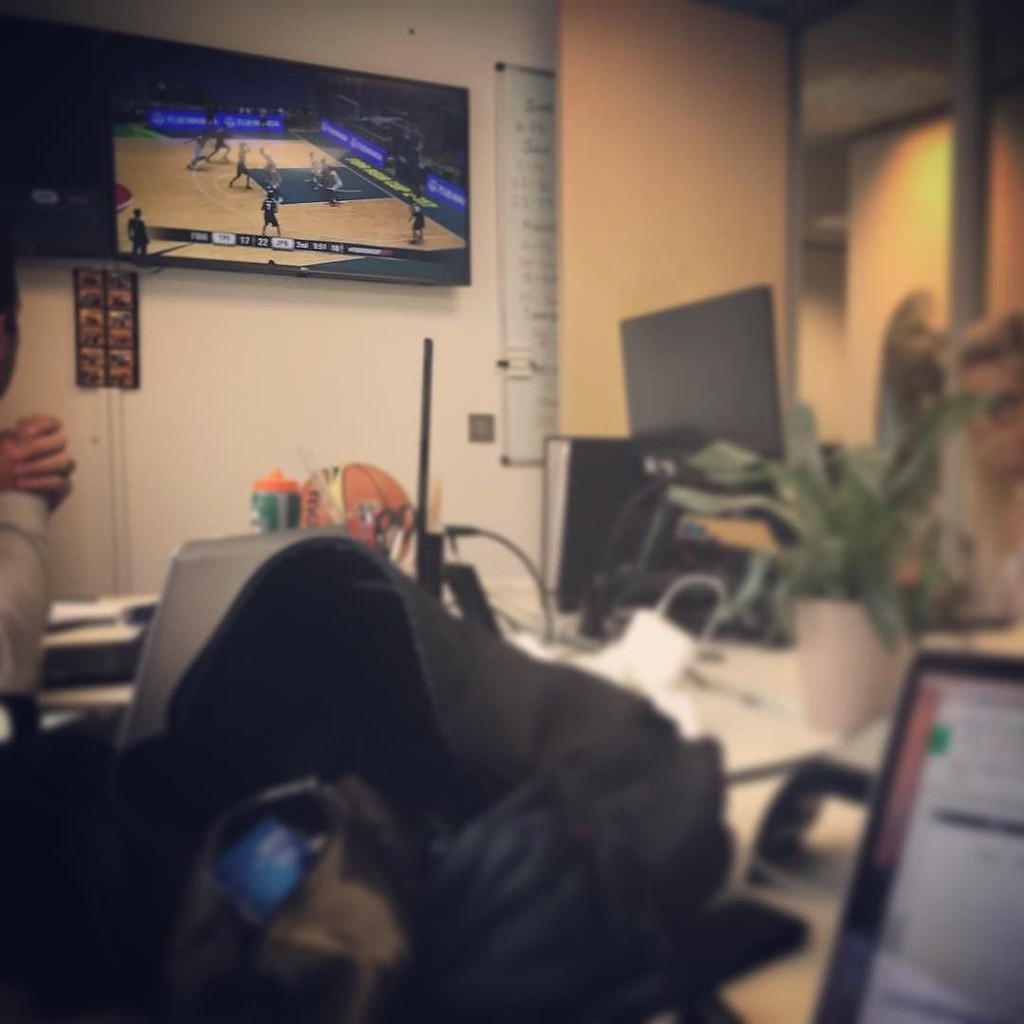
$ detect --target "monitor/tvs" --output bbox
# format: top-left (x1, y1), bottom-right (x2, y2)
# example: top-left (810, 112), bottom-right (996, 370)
top-left (0, 13), bottom-right (472, 284)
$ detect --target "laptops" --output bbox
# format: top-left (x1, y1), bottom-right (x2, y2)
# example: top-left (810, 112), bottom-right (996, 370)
top-left (807, 647), bottom-right (1024, 1024)
top-left (41, 523), bottom-right (350, 754)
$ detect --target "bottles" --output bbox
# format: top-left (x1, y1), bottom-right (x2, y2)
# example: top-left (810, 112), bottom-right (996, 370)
top-left (249, 467), bottom-right (301, 537)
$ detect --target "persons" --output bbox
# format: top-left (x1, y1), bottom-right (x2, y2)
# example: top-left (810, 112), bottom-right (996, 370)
top-left (186, 130), bottom-right (209, 170)
top-left (406, 196), bottom-right (424, 244)
top-left (228, 142), bottom-right (253, 189)
top-left (127, 207), bottom-right (150, 255)
top-left (261, 192), bottom-right (282, 238)
top-left (306, 153), bottom-right (343, 207)
top-left (258, 147), bottom-right (284, 205)
top-left (0, 207), bottom-right (75, 714)
top-left (206, 121), bottom-right (230, 161)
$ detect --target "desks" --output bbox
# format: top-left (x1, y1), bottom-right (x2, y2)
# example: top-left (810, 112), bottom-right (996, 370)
top-left (0, 594), bottom-right (1024, 1024)
